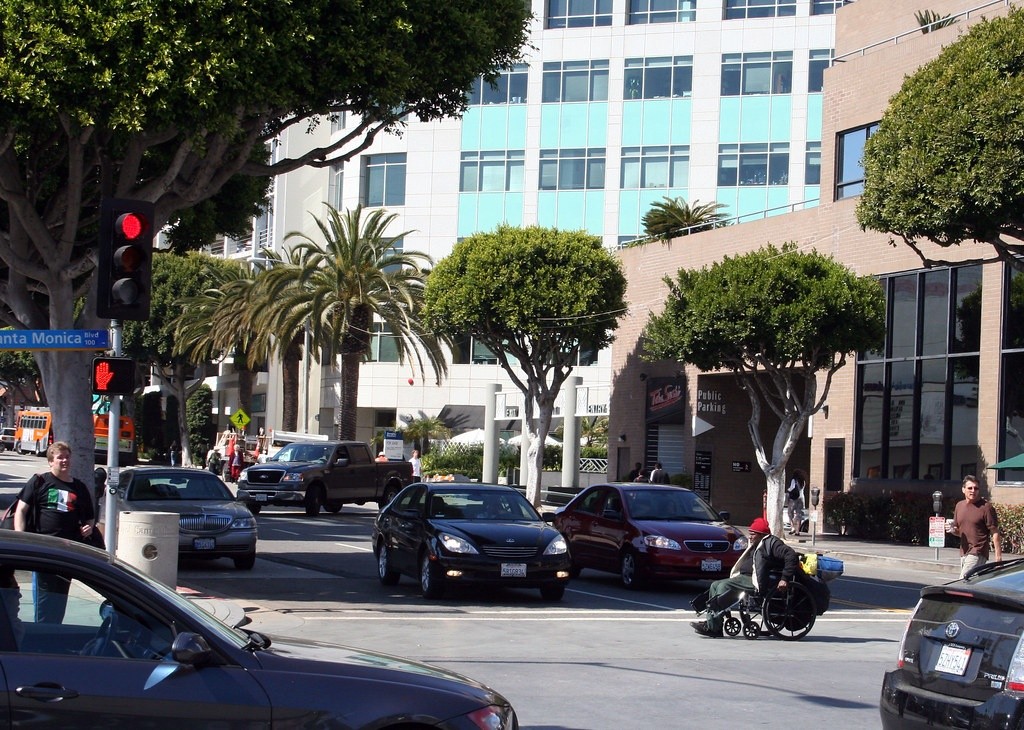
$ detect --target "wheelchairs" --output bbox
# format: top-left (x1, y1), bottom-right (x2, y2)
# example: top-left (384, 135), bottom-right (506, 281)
top-left (688, 550), bottom-right (831, 640)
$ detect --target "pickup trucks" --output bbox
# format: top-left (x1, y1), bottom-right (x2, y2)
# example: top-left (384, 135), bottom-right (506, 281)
top-left (235, 439), bottom-right (413, 518)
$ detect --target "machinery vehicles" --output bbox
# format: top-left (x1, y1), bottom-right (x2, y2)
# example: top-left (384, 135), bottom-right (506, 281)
top-left (93, 412), bottom-right (138, 467)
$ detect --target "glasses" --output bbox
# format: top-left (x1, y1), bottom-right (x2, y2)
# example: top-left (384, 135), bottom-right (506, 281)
top-left (964, 487), bottom-right (979, 490)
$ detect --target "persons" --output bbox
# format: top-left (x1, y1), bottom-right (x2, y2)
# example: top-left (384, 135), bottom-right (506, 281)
top-left (476, 500), bottom-right (509, 519)
top-left (787, 470), bottom-right (806, 536)
top-left (228, 444), bottom-right (244, 484)
top-left (628, 462), bottom-right (670, 484)
top-left (375, 452), bottom-right (389, 462)
top-left (690, 518), bottom-right (799, 638)
top-left (944, 475), bottom-right (1002, 579)
top-left (12, 441), bottom-right (95, 624)
top-left (408, 450), bottom-right (421, 483)
top-left (719, 506), bottom-right (728, 524)
top-left (170, 439), bottom-right (180, 467)
top-left (195, 445), bottom-right (221, 475)
top-left (0, 565), bottom-right (97, 666)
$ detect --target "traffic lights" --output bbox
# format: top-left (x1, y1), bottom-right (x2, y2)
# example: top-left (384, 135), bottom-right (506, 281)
top-left (91, 357), bottom-right (135, 396)
top-left (96, 197), bottom-right (156, 322)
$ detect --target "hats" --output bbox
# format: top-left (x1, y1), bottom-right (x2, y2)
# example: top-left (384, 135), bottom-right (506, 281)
top-left (749, 516), bottom-right (771, 534)
top-left (378, 451), bottom-right (386, 456)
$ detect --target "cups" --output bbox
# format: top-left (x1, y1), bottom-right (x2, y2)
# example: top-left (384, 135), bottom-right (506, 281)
top-left (946, 519), bottom-right (955, 533)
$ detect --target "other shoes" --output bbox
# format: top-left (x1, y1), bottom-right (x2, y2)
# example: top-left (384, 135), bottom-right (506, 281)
top-left (690, 622), bottom-right (723, 638)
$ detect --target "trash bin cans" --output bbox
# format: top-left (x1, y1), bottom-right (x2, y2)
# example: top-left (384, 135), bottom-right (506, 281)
top-left (117, 511), bottom-right (180, 591)
top-left (513, 467), bottom-right (520, 486)
top-left (506, 466), bottom-right (513, 485)
top-left (170, 440), bottom-right (182, 467)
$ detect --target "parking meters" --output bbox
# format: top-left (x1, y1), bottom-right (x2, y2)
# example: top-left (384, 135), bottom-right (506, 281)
top-left (811, 487), bottom-right (820, 545)
top-left (932, 491), bottom-right (944, 560)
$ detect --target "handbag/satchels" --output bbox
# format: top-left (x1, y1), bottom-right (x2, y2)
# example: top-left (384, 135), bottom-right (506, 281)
top-left (0, 474), bottom-right (41, 533)
top-left (81, 523), bottom-right (106, 550)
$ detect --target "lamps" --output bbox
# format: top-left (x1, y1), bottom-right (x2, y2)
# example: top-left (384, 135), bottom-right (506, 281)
top-left (819, 405), bottom-right (828, 413)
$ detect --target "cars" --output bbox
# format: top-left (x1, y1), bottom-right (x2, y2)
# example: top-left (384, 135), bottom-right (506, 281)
top-left (98, 464), bottom-right (257, 573)
top-left (879, 556), bottom-right (1024, 730)
top-left (371, 482), bottom-right (575, 601)
top-left (0, 428), bottom-right (16, 451)
top-left (0, 528), bottom-right (519, 730)
top-left (552, 480), bottom-right (749, 590)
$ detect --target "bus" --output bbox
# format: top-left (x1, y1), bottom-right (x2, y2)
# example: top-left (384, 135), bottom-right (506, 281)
top-left (13, 410), bottom-right (54, 456)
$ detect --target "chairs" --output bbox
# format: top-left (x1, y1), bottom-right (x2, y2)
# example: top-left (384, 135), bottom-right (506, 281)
top-left (132, 478), bottom-right (151, 497)
top-left (186, 479), bottom-right (205, 495)
